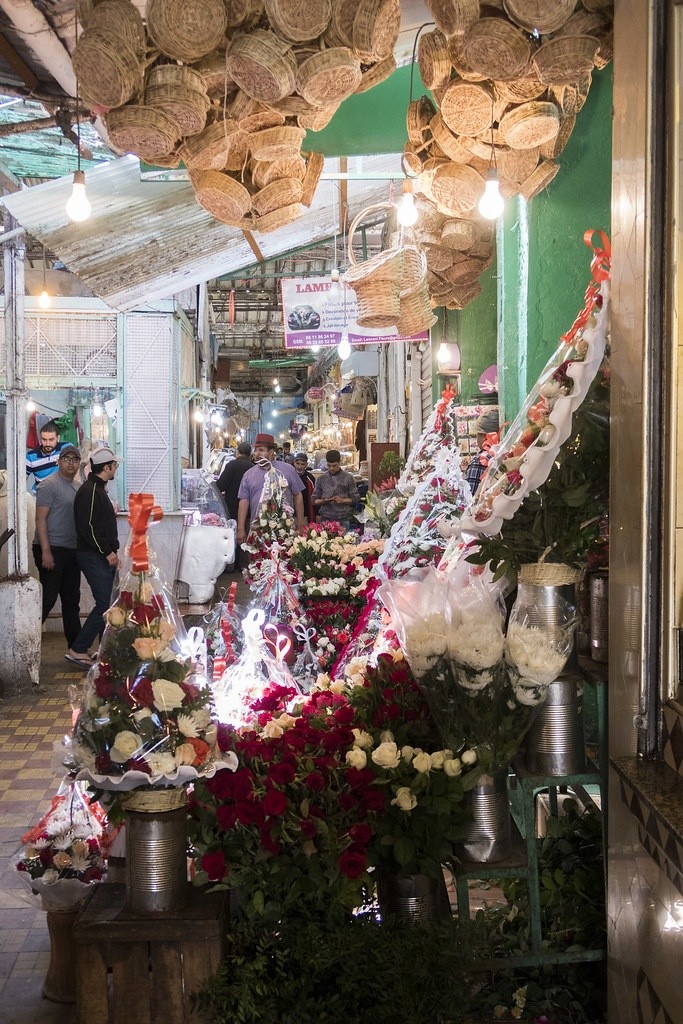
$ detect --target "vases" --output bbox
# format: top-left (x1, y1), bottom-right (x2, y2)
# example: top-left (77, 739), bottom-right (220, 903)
top-left (505, 570), bottom-right (566, 683)
top-left (527, 675), bottom-right (589, 778)
top-left (41, 911), bottom-right (80, 1004)
top-left (450, 768), bottom-right (510, 866)
top-left (120, 787), bottom-right (188, 914)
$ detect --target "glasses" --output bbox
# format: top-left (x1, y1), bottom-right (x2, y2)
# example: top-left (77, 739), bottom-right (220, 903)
top-left (60, 455), bottom-right (80, 463)
top-left (108, 463), bottom-right (119, 469)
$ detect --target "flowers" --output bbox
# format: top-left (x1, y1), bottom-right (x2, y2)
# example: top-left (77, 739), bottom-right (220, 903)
top-left (11, 280), bottom-right (612, 910)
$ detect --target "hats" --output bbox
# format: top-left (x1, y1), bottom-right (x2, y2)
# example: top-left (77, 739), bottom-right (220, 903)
top-left (89, 447), bottom-right (124, 465)
top-left (59, 446), bottom-right (81, 459)
top-left (250, 434), bottom-right (277, 447)
top-left (295, 453), bottom-right (308, 461)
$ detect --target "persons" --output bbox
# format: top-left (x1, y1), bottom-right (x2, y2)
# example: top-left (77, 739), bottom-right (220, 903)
top-left (32, 445), bottom-right (98, 661)
top-left (66, 446), bottom-right (124, 667)
top-left (216, 434), bottom-right (360, 574)
top-left (26, 422), bottom-right (75, 500)
top-left (466, 409), bottom-right (499, 498)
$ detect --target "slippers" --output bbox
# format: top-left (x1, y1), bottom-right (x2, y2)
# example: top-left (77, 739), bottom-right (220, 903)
top-left (64, 654), bottom-right (94, 668)
